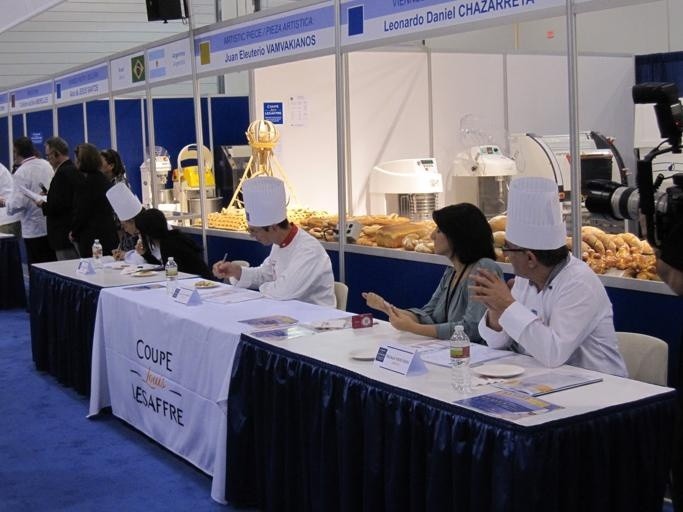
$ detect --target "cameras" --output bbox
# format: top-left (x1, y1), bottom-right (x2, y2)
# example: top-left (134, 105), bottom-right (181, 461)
top-left (585, 81), bottom-right (683, 272)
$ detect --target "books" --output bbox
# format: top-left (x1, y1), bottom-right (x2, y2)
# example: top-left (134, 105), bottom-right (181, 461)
top-left (491, 368), bottom-right (603, 397)
top-left (387, 338), bottom-right (515, 369)
top-left (200, 286), bottom-right (266, 305)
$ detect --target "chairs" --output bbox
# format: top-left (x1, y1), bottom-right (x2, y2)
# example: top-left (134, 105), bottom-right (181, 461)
top-left (225, 259), bottom-right (249, 285)
top-left (615, 331), bottom-right (667, 386)
top-left (334, 281), bottom-right (348, 311)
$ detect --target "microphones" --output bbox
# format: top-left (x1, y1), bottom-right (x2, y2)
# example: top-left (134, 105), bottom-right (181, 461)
top-left (38, 182), bottom-right (47, 193)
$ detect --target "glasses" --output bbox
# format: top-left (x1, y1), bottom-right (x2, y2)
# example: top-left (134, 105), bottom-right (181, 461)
top-left (503, 240), bottom-right (528, 255)
top-left (46, 151), bottom-right (54, 159)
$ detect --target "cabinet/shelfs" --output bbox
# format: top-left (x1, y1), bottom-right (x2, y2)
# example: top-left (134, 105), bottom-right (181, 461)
top-left (171, 222), bottom-right (682, 390)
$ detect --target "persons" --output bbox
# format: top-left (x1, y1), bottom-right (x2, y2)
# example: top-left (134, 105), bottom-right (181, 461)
top-left (69, 143), bottom-right (119, 254)
top-left (212, 177), bottom-right (337, 311)
top-left (0, 137), bottom-right (58, 264)
top-left (99, 148), bottom-right (137, 252)
top-left (106, 182), bottom-right (147, 265)
top-left (362, 203), bottom-right (506, 346)
top-left (33, 137), bottom-right (82, 261)
top-left (1, 163), bottom-right (22, 239)
top-left (467, 177), bottom-right (629, 379)
top-left (135, 208), bottom-right (213, 281)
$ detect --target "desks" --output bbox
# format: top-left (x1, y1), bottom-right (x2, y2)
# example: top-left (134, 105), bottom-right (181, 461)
top-left (0, 232), bottom-right (30, 316)
top-left (225, 320), bottom-right (676, 508)
top-left (29, 255), bottom-right (199, 399)
top-left (86, 277), bottom-right (358, 505)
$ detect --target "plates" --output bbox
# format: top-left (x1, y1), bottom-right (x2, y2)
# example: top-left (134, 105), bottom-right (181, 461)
top-left (132, 271), bottom-right (158, 276)
top-left (192, 280), bottom-right (220, 288)
top-left (472, 364), bottom-right (522, 377)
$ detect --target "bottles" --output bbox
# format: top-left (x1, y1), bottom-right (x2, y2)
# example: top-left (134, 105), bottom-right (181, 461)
top-left (449, 324), bottom-right (471, 394)
top-left (92, 239), bottom-right (103, 268)
top-left (165, 256), bottom-right (178, 297)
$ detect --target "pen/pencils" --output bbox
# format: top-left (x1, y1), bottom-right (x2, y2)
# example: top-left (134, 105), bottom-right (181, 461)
top-left (218, 252), bottom-right (228, 273)
top-left (115, 243), bottom-right (122, 262)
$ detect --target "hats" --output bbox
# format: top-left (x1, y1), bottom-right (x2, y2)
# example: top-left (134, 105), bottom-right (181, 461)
top-left (242, 177), bottom-right (285, 227)
top-left (107, 183), bottom-right (143, 220)
top-left (505, 176), bottom-right (566, 249)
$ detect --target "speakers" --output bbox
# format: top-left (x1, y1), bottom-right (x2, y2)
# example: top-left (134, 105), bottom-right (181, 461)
top-left (145, 0), bottom-right (189, 24)
